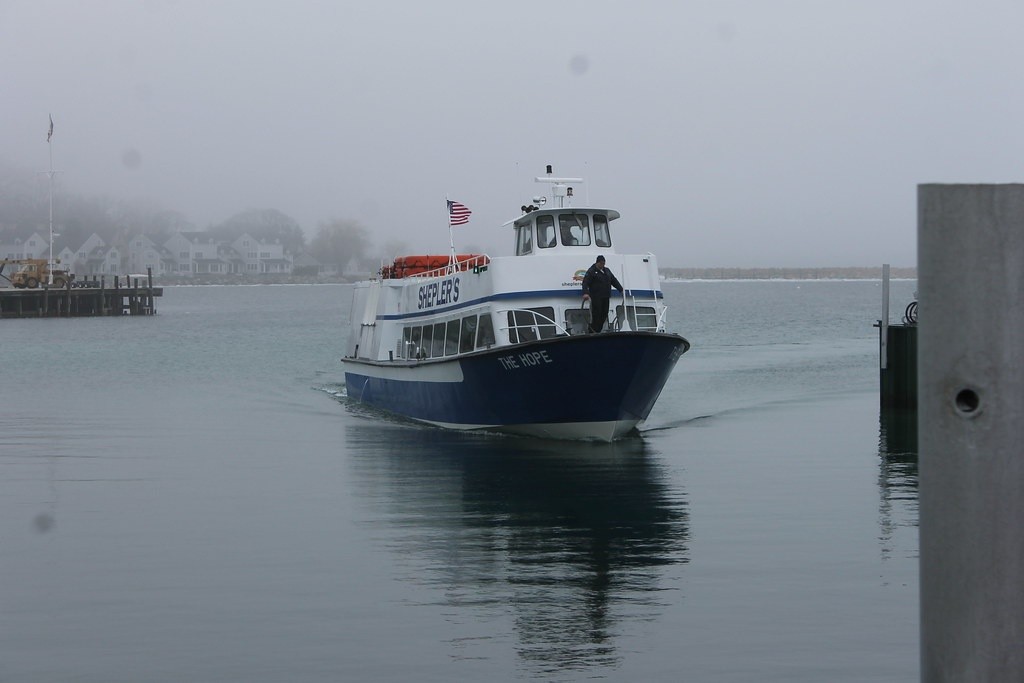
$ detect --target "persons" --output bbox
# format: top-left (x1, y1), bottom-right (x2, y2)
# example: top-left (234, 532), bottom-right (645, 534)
top-left (581, 254), bottom-right (623, 332)
top-left (547, 224), bottom-right (573, 247)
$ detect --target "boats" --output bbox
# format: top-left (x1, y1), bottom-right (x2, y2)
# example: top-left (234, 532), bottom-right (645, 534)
top-left (341, 173), bottom-right (691, 443)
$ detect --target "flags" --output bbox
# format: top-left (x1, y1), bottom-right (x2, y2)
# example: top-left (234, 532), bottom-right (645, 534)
top-left (46, 114), bottom-right (54, 142)
top-left (446, 199), bottom-right (471, 225)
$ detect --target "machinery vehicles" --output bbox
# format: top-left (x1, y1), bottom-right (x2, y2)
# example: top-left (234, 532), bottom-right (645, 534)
top-left (0, 258), bottom-right (75, 288)
